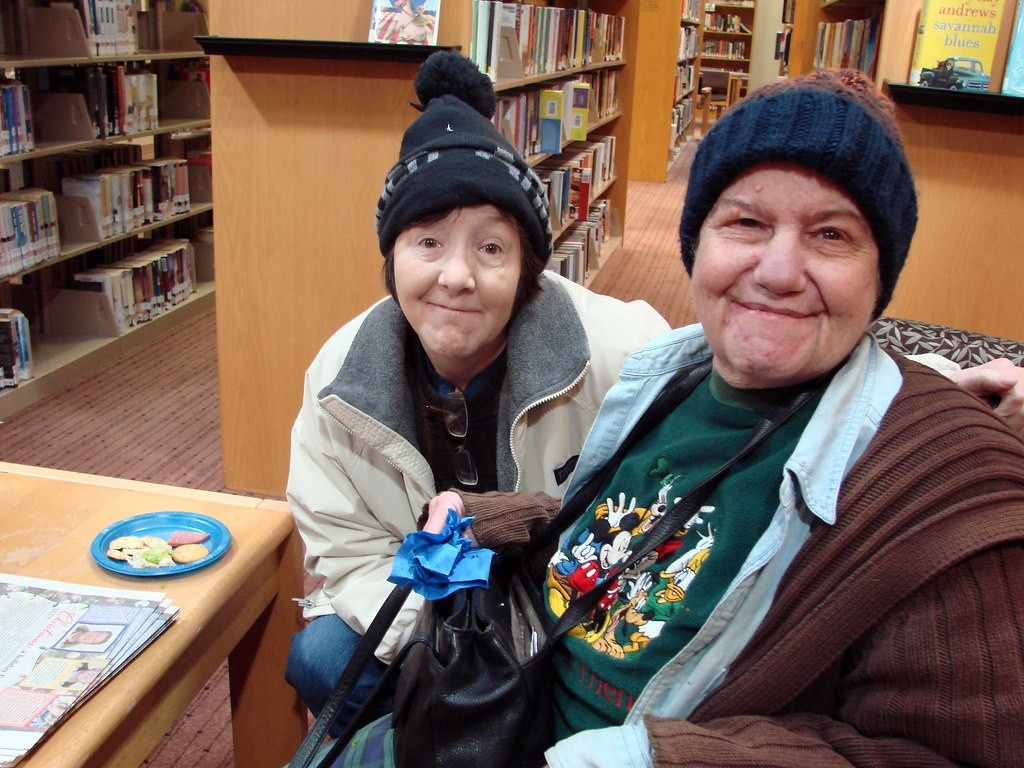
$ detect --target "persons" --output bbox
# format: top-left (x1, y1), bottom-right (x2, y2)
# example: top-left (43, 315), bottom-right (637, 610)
top-left (280, 98), bottom-right (1024, 767)
top-left (291, 70), bottom-right (1024, 768)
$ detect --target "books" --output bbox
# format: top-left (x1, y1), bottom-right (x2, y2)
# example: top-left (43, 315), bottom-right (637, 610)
top-left (1000, 0), bottom-right (1024, 98)
top-left (468, 1), bottom-right (626, 289)
top-left (667, 0), bottom-right (886, 158)
top-left (367, 1), bottom-right (442, 46)
top-left (908, 0), bottom-right (1005, 95)
top-left (0, 0), bottom-right (203, 386)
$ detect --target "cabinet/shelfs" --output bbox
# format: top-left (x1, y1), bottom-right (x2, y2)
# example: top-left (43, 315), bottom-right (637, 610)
top-left (193, 1), bottom-right (627, 498)
top-left (0, 0), bottom-right (215, 422)
top-left (788, 0), bottom-right (1023, 345)
top-left (624, 0), bottom-right (706, 185)
top-left (695, 0), bottom-right (755, 121)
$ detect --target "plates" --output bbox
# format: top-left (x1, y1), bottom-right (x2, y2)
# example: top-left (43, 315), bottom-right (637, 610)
top-left (91, 512), bottom-right (231, 576)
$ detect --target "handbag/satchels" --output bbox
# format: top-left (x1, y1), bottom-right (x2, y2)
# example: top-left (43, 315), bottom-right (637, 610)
top-left (391, 536), bottom-right (559, 768)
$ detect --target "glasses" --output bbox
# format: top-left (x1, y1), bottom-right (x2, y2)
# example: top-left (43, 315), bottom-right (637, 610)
top-left (425, 388), bottom-right (479, 488)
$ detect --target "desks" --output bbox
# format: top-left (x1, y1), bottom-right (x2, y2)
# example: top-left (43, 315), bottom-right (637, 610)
top-left (1, 461), bottom-right (310, 768)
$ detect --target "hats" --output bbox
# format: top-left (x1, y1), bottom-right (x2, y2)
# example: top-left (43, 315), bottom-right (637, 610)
top-left (373, 50), bottom-right (550, 273)
top-left (678, 67), bottom-right (919, 322)
